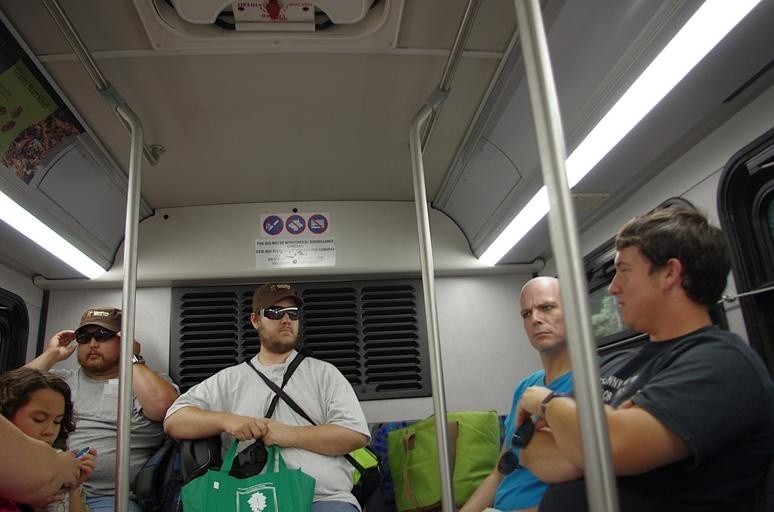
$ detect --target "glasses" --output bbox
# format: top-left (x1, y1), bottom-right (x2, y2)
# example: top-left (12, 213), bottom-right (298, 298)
top-left (498, 419), bottom-right (534, 475)
top-left (74, 329), bottom-right (116, 345)
top-left (264, 306), bottom-right (301, 321)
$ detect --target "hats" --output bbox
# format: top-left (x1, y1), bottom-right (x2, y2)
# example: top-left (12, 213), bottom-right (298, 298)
top-left (75, 308), bottom-right (121, 335)
top-left (252, 284), bottom-right (303, 314)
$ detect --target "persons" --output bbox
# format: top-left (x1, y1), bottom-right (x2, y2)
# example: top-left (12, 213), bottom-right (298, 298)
top-left (161, 283), bottom-right (372, 511)
top-left (25, 306), bottom-right (182, 511)
top-left (515, 206), bottom-right (774, 512)
top-left (0, 415), bottom-right (83, 512)
top-left (457, 276), bottom-right (575, 512)
top-left (0, 365), bottom-right (98, 511)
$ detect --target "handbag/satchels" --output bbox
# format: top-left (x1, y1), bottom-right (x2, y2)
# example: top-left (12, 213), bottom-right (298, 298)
top-left (182, 470), bottom-right (315, 512)
top-left (132, 438), bottom-right (219, 512)
top-left (350, 447), bottom-right (379, 498)
top-left (387, 412), bottom-right (500, 511)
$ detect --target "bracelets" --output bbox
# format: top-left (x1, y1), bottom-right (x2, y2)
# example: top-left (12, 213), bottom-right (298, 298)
top-left (541, 391), bottom-right (569, 415)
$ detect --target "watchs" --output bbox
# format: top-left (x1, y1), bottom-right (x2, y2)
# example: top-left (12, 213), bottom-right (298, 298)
top-left (132, 354), bottom-right (146, 363)
